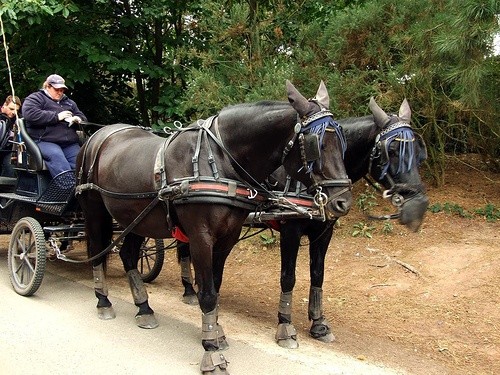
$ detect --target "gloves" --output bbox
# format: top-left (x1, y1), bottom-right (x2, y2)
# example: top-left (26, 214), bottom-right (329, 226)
top-left (66, 116), bottom-right (82, 127)
top-left (58, 110), bottom-right (72, 120)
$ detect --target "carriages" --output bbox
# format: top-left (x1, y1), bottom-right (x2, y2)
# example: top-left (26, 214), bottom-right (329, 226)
top-left (0, 79), bottom-right (430, 375)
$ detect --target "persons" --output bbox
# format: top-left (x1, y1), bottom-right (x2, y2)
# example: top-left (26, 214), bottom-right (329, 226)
top-left (0, 94), bottom-right (23, 179)
top-left (21, 74), bottom-right (92, 194)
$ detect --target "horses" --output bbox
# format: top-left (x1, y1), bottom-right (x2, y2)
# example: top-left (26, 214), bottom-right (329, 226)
top-left (75, 79), bottom-right (352, 351)
top-left (178, 96), bottom-right (429, 350)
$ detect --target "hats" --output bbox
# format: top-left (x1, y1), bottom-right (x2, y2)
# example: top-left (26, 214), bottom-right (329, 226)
top-left (47, 74), bottom-right (68, 89)
